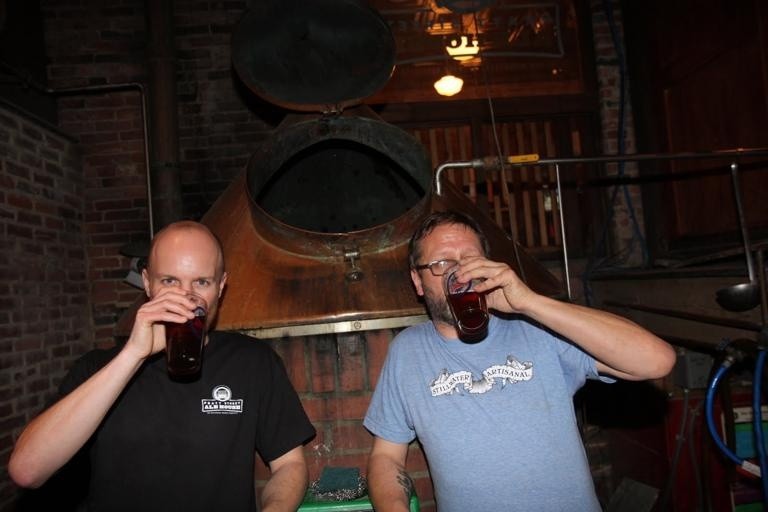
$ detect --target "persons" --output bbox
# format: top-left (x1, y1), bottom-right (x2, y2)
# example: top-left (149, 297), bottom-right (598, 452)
top-left (9, 221), bottom-right (310, 512)
top-left (368, 211), bottom-right (676, 512)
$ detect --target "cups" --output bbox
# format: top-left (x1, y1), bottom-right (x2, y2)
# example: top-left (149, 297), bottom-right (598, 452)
top-left (443, 269), bottom-right (490, 335)
top-left (163, 293), bottom-right (209, 376)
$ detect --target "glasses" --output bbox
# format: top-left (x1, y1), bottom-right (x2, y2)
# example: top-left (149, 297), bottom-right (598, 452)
top-left (415, 255), bottom-right (469, 277)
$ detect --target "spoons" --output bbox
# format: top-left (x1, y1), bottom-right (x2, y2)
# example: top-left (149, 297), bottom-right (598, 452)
top-left (714, 162), bottom-right (764, 313)
